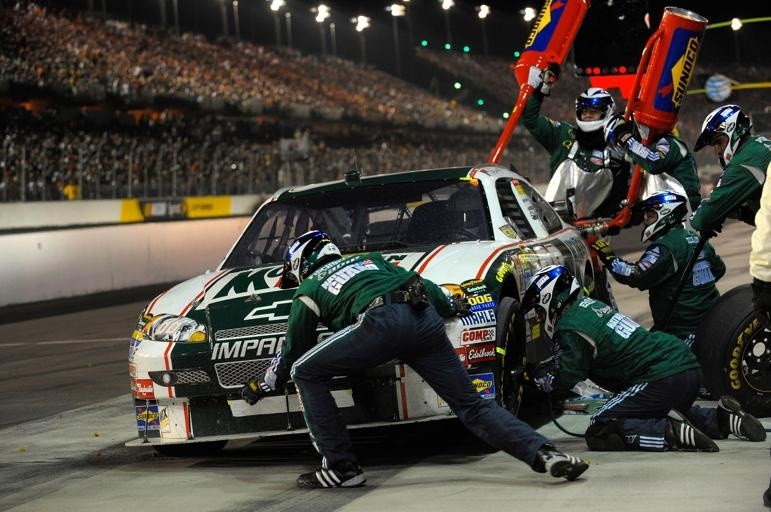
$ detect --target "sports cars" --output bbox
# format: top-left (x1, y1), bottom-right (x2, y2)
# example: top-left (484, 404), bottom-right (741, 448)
top-left (126, 165), bottom-right (608, 450)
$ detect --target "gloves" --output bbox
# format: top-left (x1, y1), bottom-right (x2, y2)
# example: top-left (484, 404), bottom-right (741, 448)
top-left (448, 291), bottom-right (473, 319)
top-left (238, 376), bottom-right (270, 406)
top-left (615, 130), bottom-right (637, 150)
top-left (538, 61), bottom-right (562, 95)
top-left (705, 222), bottom-right (724, 239)
top-left (533, 372), bottom-right (566, 395)
top-left (591, 239), bottom-right (617, 264)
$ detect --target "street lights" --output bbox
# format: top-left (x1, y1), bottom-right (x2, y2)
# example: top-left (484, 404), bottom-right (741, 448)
top-left (440, 0), bottom-right (457, 46)
top-left (351, 14), bottom-right (372, 67)
top-left (730, 17), bottom-right (744, 67)
top-left (228, 0), bottom-right (341, 60)
top-left (387, 3), bottom-right (406, 77)
top-left (477, 4), bottom-right (493, 58)
top-left (402, 0), bottom-right (416, 46)
top-left (520, 7), bottom-right (538, 32)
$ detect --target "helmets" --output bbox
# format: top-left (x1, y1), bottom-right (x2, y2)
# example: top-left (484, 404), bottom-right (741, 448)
top-left (278, 229), bottom-right (342, 291)
top-left (519, 264), bottom-right (580, 341)
top-left (693, 103), bottom-right (751, 169)
top-left (638, 189), bottom-right (688, 244)
top-left (574, 87), bottom-right (616, 133)
top-left (602, 111), bottom-right (644, 153)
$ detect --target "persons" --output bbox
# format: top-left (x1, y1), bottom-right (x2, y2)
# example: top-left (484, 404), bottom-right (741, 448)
top-left (689, 103), bottom-right (771, 240)
top-left (513, 263), bottom-right (767, 454)
top-left (1, 1), bottom-right (585, 205)
top-left (519, 61), bottom-right (631, 313)
top-left (586, 110), bottom-right (702, 240)
top-left (747, 159), bottom-right (771, 509)
top-left (589, 189), bottom-right (725, 400)
top-left (239, 229), bottom-right (592, 490)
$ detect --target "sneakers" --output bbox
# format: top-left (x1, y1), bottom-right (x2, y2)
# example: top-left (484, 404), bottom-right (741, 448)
top-left (667, 406), bottom-right (721, 452)
top-left (294, 464), bottom-right (367, 491)
top-left (537, 449), bottom-right (589, 481)
top-left (717, 394), bottom-right (767, 442)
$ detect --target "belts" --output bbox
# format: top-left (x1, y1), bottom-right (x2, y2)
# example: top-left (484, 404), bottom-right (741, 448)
top-left (365, 288), bottom-right (417, 308)
